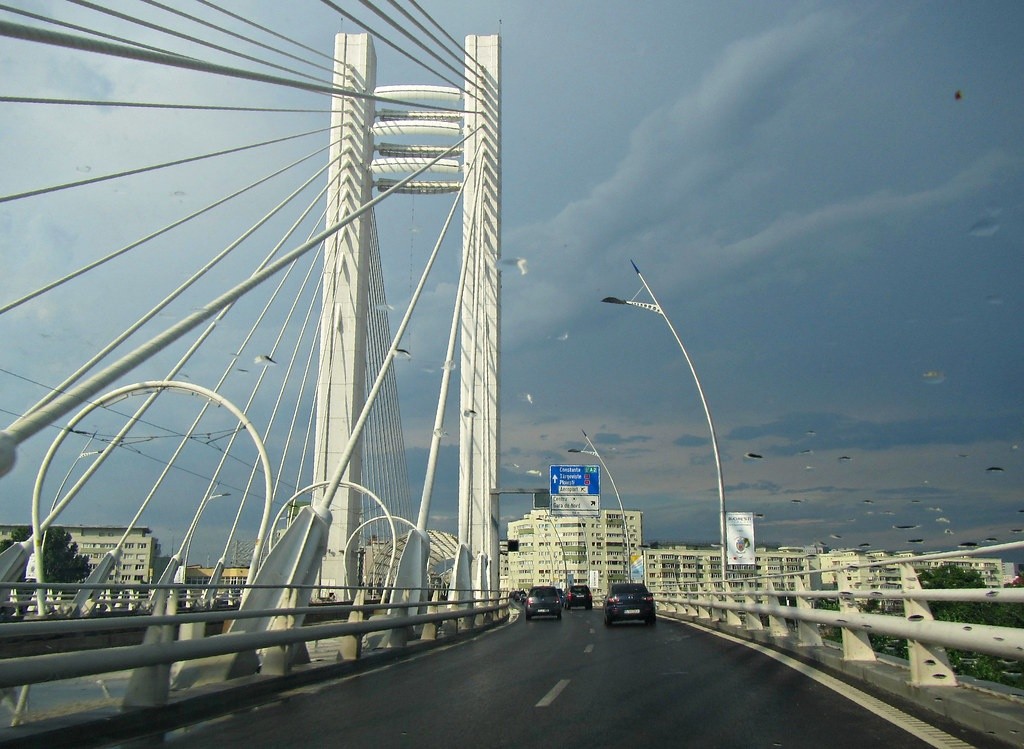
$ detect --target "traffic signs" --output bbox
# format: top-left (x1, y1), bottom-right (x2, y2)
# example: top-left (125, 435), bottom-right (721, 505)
top-left (549, 465), bottom-right (600, 518)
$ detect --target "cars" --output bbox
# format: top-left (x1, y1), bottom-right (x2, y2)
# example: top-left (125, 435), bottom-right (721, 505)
top-left (558, 588), bottom-right (564, 604)
top-left (509, 591), bottom-right (527, 603)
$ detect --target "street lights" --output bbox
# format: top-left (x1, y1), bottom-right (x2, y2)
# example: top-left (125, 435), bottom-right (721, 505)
top-left (601, 298), bottom-right (728, 593)
top-left (40, 449), bottom-right (104, 553)
top-left (179, 492), bottom-right (231, 584)
top-left (567, 449), bottom-right (634, 585)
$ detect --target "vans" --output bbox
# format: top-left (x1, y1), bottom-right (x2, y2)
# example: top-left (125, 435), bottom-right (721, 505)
top-left (524, 586), bottom-right (561, 618)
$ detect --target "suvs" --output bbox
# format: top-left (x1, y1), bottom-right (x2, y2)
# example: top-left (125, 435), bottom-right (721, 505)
top-left (565, 584), bottom-right (593, 609)
top-left (603, 584), bottom-right (656, 625)
